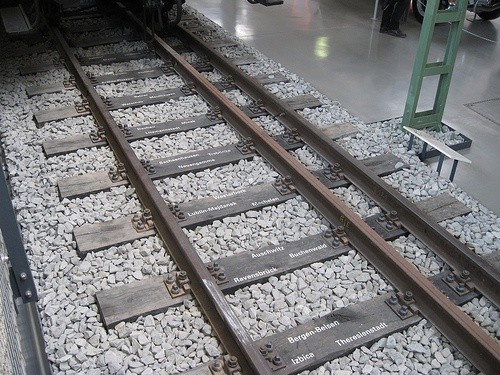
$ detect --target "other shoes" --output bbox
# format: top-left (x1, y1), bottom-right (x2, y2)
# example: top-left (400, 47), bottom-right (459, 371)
top-left (380, 27), bottom-right (407, 38)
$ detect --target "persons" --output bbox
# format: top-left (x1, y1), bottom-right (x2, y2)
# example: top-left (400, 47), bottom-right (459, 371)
top-left (379, 0), bottom-right (408, 38)
